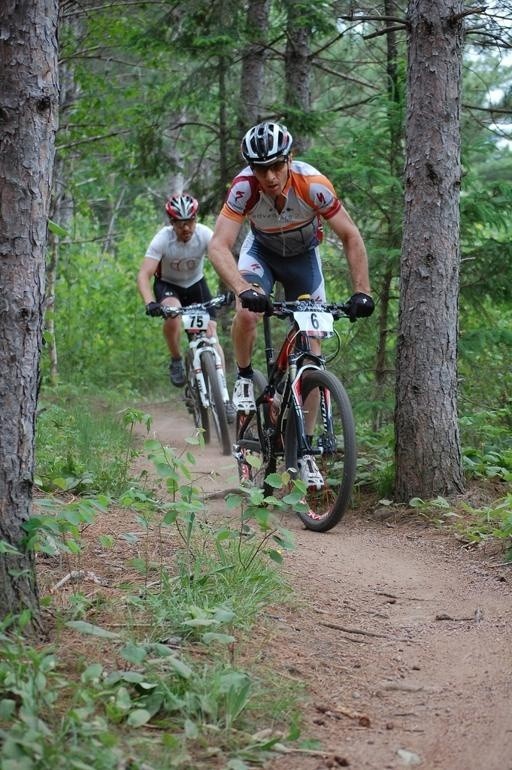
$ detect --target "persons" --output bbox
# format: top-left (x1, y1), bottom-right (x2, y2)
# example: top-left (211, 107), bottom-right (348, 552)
top-left (137, 193), bottom-right (239, 429)
top-left (200, 110), bottom-right (374, 493)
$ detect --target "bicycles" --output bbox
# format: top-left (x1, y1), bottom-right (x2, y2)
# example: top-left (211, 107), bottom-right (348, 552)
top-left (145, 291), bottom-right (239, 459)
top-left (234, 290), bottom-right (365, 530)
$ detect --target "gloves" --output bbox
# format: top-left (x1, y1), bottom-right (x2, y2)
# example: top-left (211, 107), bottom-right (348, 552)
top-left (145, 301), bottom-right (163, 318)
top-left (345, 291), bottom-right (375, 322)
top-left (236, 283), bottom-right (274, 316)
top-left (222, 291), bottom-right (236, 306)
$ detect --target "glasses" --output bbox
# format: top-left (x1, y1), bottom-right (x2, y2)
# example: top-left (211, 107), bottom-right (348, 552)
top-left (174, 219), bottom-right (194, 230)
top-left (252, 159), bottom-right (285, 178)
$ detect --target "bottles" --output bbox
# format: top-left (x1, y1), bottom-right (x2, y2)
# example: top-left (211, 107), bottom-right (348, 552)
top-left (269, 381), bottom-right (286, 428)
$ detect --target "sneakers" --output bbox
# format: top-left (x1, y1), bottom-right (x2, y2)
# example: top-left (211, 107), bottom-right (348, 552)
top-left (232, 376), bottom-right (257, 416)
top-left (222, 399), bottom-right (235, 423)
top-left (169, 356), bottom-right (187, 387)
top-left (298, 455), bottom-right (324, 491)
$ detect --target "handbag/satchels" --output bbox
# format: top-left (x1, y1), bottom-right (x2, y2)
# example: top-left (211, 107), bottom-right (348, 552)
top-left (254, 224), bottom-right (321, 258)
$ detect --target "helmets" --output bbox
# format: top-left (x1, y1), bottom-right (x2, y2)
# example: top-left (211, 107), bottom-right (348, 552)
top-left (239, 120), bottom-right (293, 165)
top-left (165, 192), bottom-right (199, 221)
